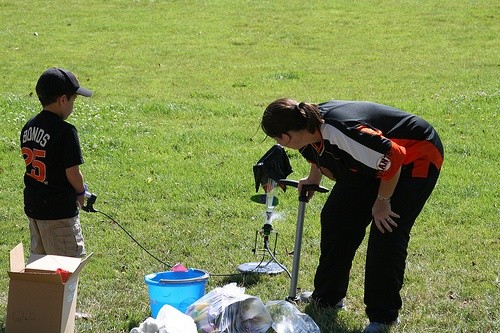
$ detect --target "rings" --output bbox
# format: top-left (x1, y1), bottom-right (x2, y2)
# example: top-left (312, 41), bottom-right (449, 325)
top-left (386, 215), bottom-right (390, 220)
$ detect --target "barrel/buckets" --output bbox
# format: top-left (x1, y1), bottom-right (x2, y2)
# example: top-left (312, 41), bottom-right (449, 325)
top-left (144, 268), bottom-right (209, 319)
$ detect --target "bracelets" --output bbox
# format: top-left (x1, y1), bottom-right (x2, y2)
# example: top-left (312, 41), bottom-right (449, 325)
top-left (377, 193), bottom-right (393, 201)
top-left (75, 189), bottom-right (86, 197)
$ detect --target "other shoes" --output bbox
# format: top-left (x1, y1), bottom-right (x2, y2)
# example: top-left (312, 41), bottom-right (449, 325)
top-left (302, 292), bottom-right (346, 309)
top-left (363, 317), bottom-right (401, 333)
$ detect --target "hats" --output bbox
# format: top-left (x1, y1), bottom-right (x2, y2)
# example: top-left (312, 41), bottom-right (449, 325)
top-left (36, 68), bottom-right (92, 97)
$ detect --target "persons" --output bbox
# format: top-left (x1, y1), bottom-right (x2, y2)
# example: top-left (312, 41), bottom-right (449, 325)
top-left (260, 97), bottom-right (444, 333)
top-left (19, 67), bottom-right (93, 260)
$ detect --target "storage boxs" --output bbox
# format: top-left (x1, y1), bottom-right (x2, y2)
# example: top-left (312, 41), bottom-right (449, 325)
top-left (6, 241), bottom-right (94, 333)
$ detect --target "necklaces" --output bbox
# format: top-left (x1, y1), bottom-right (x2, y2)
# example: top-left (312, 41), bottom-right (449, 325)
top-left (313, 142), bottom-right (323, 152)
